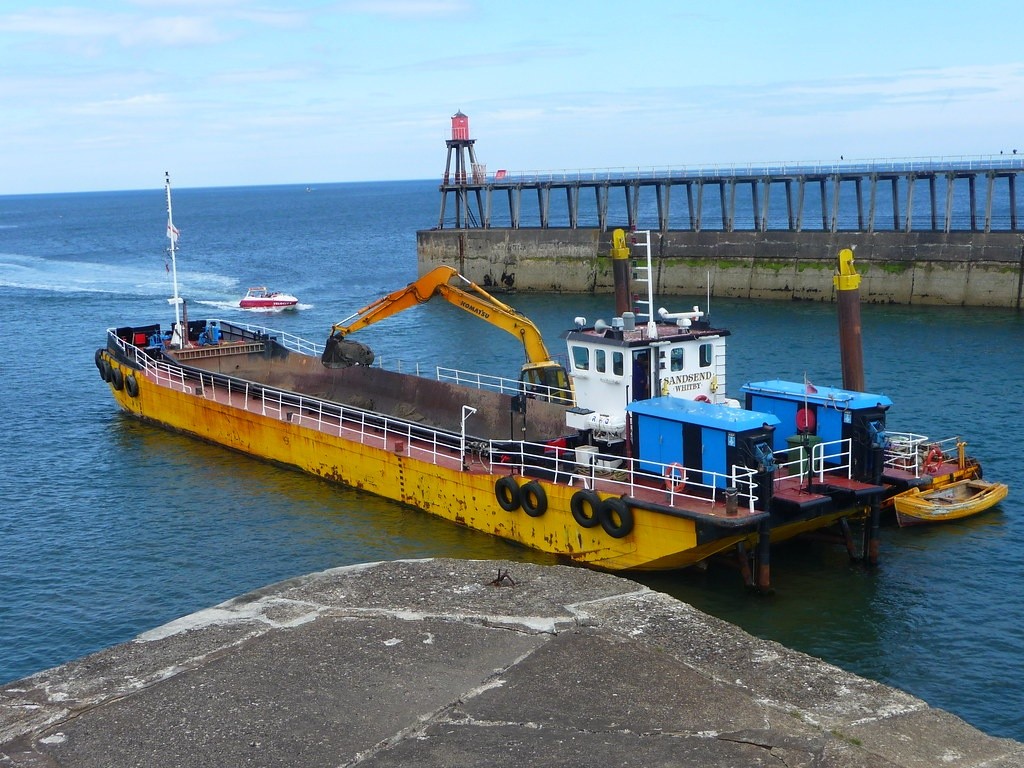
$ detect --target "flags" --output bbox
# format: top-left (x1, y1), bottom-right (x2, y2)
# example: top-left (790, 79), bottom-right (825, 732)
top-left (166, 219), bottom-right (179, 243)
top-left (805, 379), bottom-right (818, 394)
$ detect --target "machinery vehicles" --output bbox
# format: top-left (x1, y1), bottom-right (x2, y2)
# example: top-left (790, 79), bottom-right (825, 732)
top-left (318, 264), bottom-right (576, 406)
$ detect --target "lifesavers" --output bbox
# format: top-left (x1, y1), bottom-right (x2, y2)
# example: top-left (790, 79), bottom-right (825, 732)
top-left (495, 477), bottom-right (520, 512)
top-left (665, 463), bottom-right (686, 492)
top-left (100, 361), bottom-right (111, 383)
top-left (519, 481), bottom-right (547, 517)
top-left (111, 368), bottom-right (123, 391)
top-left (570, 489), bottom-right (601, 528)
top-left (94, 348), bottom-right (102, 369)
top-left (927, 448), bottom-right (942, 473)
top-left (694, 395), bottom-right (711, 404)
top-left (125, 374), bottom-right (138, 398)
top-left (598, 496), bottom-right (633, 538)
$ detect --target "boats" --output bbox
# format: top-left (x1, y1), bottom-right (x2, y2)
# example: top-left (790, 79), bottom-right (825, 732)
top-left (95, 171), bottom-right (984, 576)
top-left (238, 286), bottom-right (301, 313)
top-left (892, 475), bottom-right (1010, 521)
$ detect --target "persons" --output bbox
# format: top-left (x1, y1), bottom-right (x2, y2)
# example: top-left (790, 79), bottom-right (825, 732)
top-left (536, 381), bottom-right (544, 391)
top-left (261, 292), bottom-right (277, 297)
top-left (634, 353), bottom-right (645, 400)
